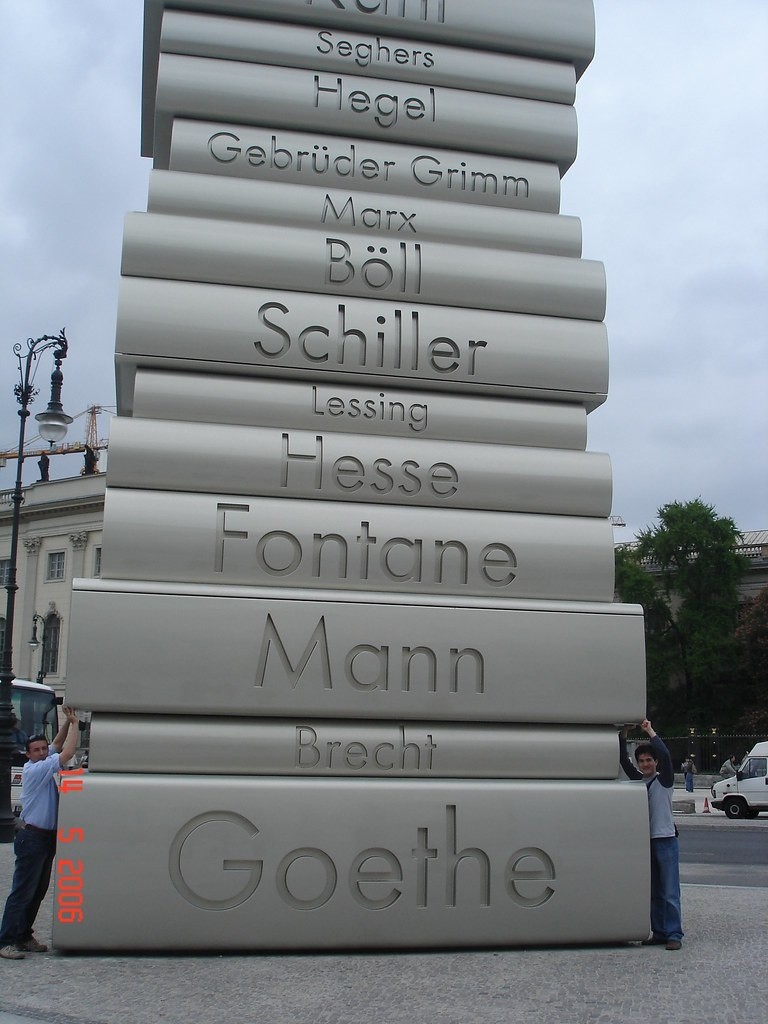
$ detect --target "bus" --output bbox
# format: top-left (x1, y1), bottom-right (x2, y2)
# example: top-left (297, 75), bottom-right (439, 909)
top-left (10, 679), bottom-right (63, 823)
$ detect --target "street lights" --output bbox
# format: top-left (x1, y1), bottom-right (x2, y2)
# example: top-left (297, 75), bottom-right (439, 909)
top-left (0, 327), bottom-right (69, 845)
top-left (26, 613), bottom-right (47, 684)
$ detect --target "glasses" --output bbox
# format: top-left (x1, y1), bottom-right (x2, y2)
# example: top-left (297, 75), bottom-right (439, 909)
top-left (26, 734), bottom-right (43, 746)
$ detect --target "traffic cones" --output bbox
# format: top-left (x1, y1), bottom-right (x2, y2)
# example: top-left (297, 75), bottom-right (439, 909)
top-left (702, 797), bottom-right (711, 813)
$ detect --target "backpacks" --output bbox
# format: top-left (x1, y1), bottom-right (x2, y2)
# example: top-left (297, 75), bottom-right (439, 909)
top-left (692, 763), bottom-right (696, 773)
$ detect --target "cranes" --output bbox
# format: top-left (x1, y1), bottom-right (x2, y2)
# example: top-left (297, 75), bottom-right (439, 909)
top-left (0, 405), bottom-right (119, 477)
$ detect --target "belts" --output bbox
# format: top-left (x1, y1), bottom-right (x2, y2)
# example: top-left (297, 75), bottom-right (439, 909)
top-left (26, 825), bottom-right (57, 835)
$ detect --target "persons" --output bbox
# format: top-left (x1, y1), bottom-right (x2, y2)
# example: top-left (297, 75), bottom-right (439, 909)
top-left (681, 753), bottom-right (697, 792)
top-left (618, 718), bottom-right (685, 951)
top-left (0, 704), bottom-right (79, 960)
top-left (9, 719), bottom-right (28, 752)
top-left (719, 755), bottom-right (737, 779)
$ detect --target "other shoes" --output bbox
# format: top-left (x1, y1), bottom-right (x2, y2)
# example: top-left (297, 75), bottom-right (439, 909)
top-left (16, 938), bottom-right (47, 952)
top-left (666, 940), bottom-right (681, 950)
top-left (641, 936), bottom-right (664, 945)
top-left (0, 945), bottom-right (25, 959)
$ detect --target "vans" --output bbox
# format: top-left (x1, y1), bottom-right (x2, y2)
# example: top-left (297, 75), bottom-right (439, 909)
top-left (710, 741), bottom-right (768, 820)
